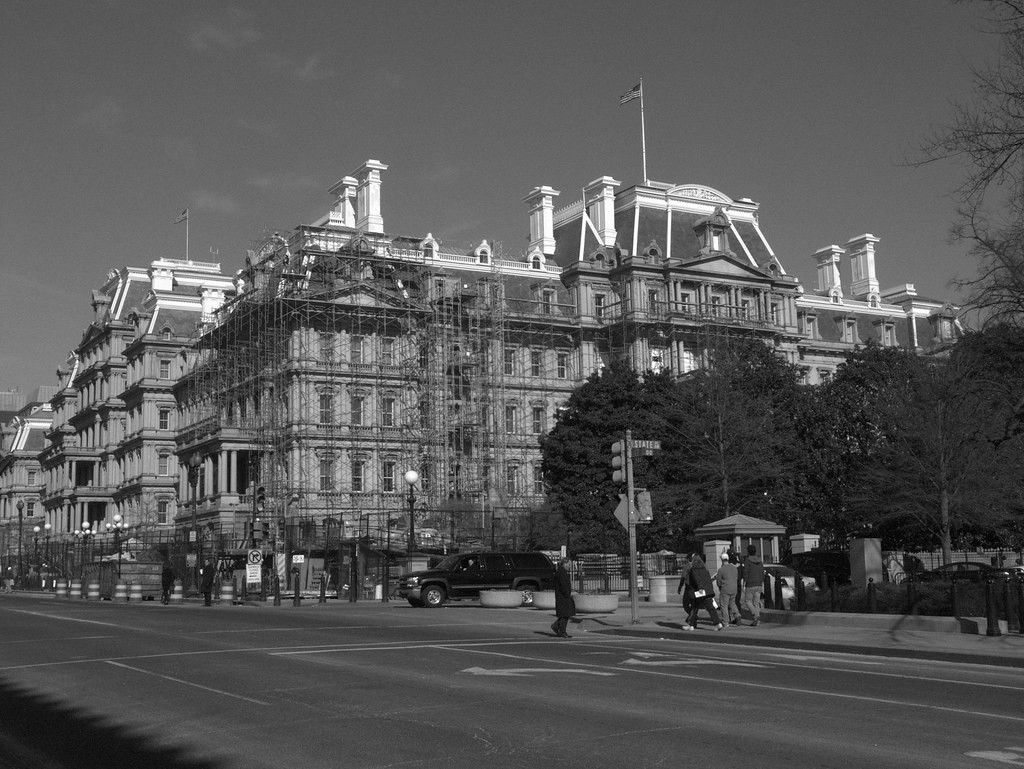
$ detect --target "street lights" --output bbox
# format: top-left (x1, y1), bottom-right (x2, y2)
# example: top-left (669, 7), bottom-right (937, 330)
top-left (403, 470), bottom-right (420, 550)
top-left (187, 450), bottom-right (202, 593)
top-left (33, 525), bottom-right (41, 558)
top-left (44, 523), bottom-right (52, 557)
top-left (16, 500), bottom-right (25, 569)
top-left (105, 514), bottom-right (130, 553)
top-left (74, 521), bottom-right (98, 551)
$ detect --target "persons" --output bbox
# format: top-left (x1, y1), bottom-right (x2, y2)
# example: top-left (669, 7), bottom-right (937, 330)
top-left (744, 545), bottom-right (765, 626)
top-left (677, 553), bottom-right (724, 631)
top-left (551, 557), bottom-right (575, 639)
top-left (717, 544), bottom-right (743, 628)
top-left (463, 560), bottom-right (480, 572)
top-left (160, 563), bottom-right (172, 605)
top-left (201, 559), bottom-right (214, 606)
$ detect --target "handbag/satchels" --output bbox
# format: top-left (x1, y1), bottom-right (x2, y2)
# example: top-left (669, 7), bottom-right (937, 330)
top-left (711, 598), bottom-right (718, 609)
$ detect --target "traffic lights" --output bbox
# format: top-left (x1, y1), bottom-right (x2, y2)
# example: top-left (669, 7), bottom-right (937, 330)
top-left (257, 486), bottom-right (266, 512)
top-left (611, 438), bottom-right (626, 483)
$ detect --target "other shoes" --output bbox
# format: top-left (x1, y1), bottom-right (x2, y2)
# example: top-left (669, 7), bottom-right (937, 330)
top-left (718, 623), bottom-right (723, 631)
top-left (751, 616), bottom-right (761, 626)
top-left (736, 616), bottom-right (742, 625)
top-left (682, 624), bottom-right (694, 630)
top-left (557, 633), bottom-right (572, 638)
top-left (551, 625), bottom-right (558, 634)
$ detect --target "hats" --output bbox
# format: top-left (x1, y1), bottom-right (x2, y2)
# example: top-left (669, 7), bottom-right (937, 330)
top-left (721, 553), bottom-right (729, 560)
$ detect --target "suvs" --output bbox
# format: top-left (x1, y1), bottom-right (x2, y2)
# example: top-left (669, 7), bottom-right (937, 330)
top-left (398, 551), bottom-right (558, 609)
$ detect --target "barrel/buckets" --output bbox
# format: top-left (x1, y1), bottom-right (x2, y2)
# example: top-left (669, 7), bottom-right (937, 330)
top-left (116, 579), bottom-right (142, 602)
top-left (88, 580), bottom-right (99, 601)
top-left (221, 580), bottom-right (234, 606)
top-left (56, 580), bottom-right (67, 599)
top-left (70, 579), bottom-right (81, 600)
top-left (167, 579), bottom-right (183, 605)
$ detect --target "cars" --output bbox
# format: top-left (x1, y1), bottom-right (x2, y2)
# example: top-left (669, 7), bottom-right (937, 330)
top-left (900, 560), bottom-right (1011, 585)
top-left (713, 564), bottom-right (820, 595)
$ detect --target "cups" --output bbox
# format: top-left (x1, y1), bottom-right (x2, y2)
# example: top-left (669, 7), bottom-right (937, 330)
top-left (200, 569), bottom-right (204, 574)
